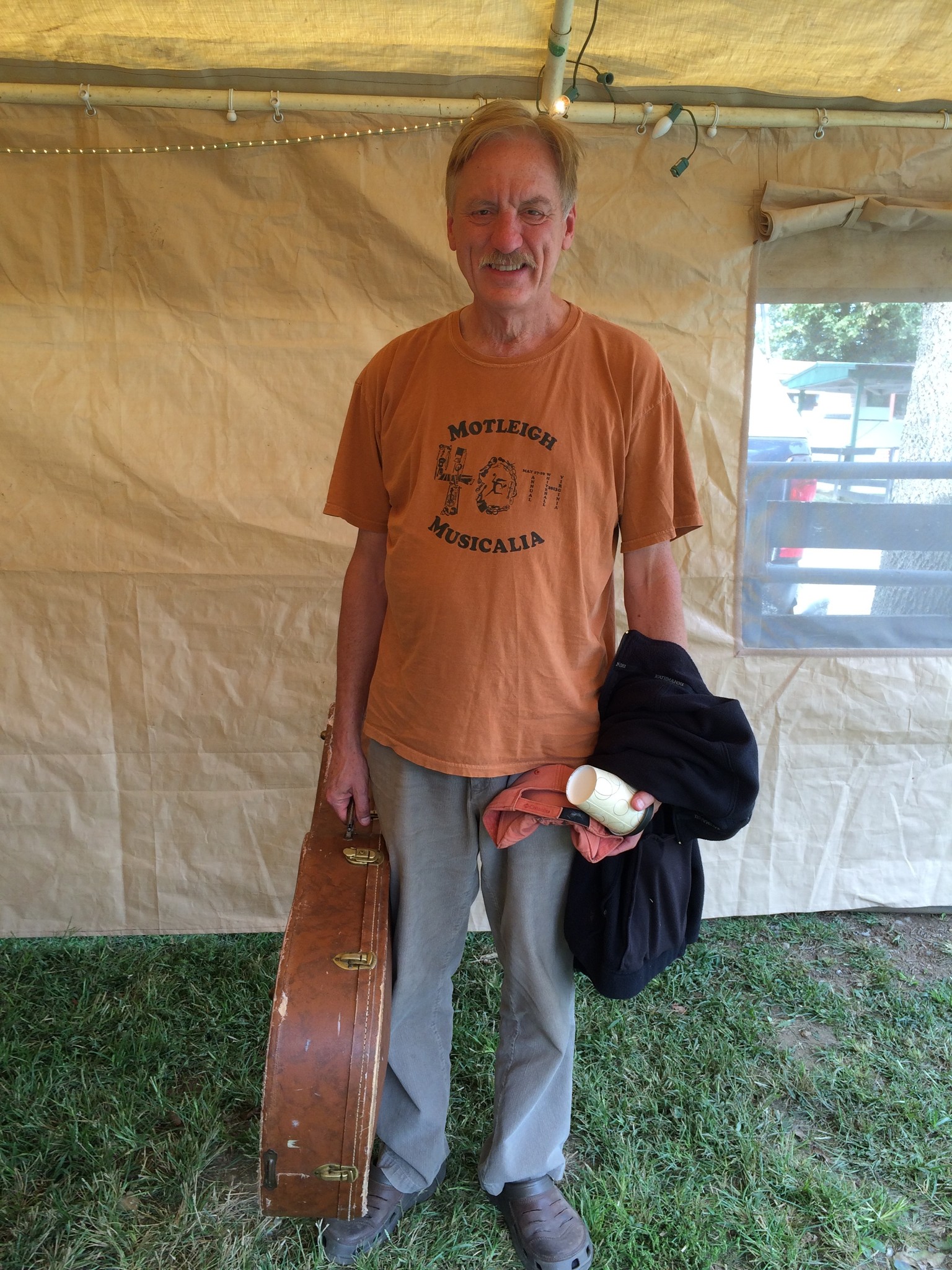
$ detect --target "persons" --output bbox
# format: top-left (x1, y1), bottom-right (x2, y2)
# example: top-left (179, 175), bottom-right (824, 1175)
top-left (319, 100), bottom-right (705, 1266)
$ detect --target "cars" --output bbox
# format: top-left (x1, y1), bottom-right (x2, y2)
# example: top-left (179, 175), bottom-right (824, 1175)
top-left (742, 346), bottom-right (816, 616)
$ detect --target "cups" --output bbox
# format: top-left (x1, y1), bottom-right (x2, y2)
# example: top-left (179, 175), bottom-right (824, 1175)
top-left (566, 766), bottom-right (654, 837)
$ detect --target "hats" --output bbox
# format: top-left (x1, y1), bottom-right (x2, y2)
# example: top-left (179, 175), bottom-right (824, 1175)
top-left (482, 764), bottom-right (624, 863)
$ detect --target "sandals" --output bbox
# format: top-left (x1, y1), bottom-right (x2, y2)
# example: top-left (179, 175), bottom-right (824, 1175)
top-left (320, 1157), bottom-right (446, 1265)
top-left (497, 1173), bottom-right (594, 1270)
